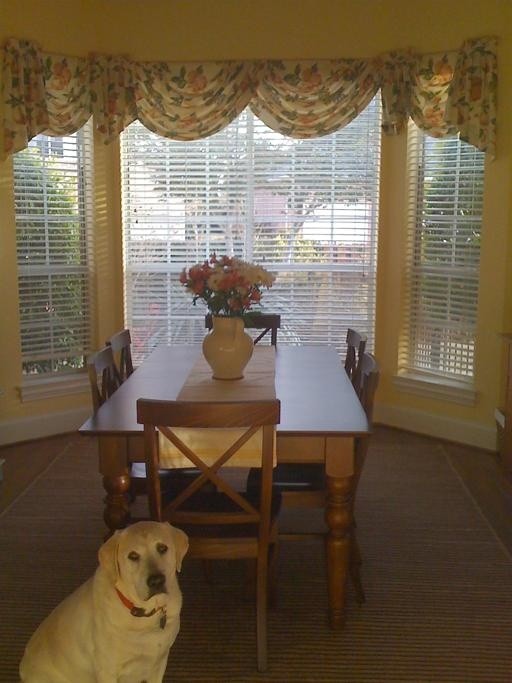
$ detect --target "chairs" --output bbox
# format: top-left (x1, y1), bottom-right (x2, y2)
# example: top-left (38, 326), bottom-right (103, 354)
top-left (85, 345), bottom-right (222, 600)
top-left (128, 395), bottom-right (272, 672)
top-left (242, 350), bottom-right (380, 611)
top-left (338, 327), bottom-right (370, 380)
top-left (200, 311), bottom-right (283, 349)
top-left (103, 327), bottom-right (141, 373)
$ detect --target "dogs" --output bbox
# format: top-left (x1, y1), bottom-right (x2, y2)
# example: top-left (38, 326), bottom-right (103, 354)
top-left (18, 521), bottom-right (190, 683)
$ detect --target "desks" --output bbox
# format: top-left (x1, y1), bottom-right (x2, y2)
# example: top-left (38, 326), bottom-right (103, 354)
top-left (78, 343), bottom-right (373, 632)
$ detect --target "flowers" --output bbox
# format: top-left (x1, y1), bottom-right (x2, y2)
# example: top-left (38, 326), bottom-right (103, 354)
top-left (181, 254), bottom-right (274, 331)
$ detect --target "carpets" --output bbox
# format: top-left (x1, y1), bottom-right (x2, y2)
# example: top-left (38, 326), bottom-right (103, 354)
top-left (3, 436), bottom-right (510, 682)
